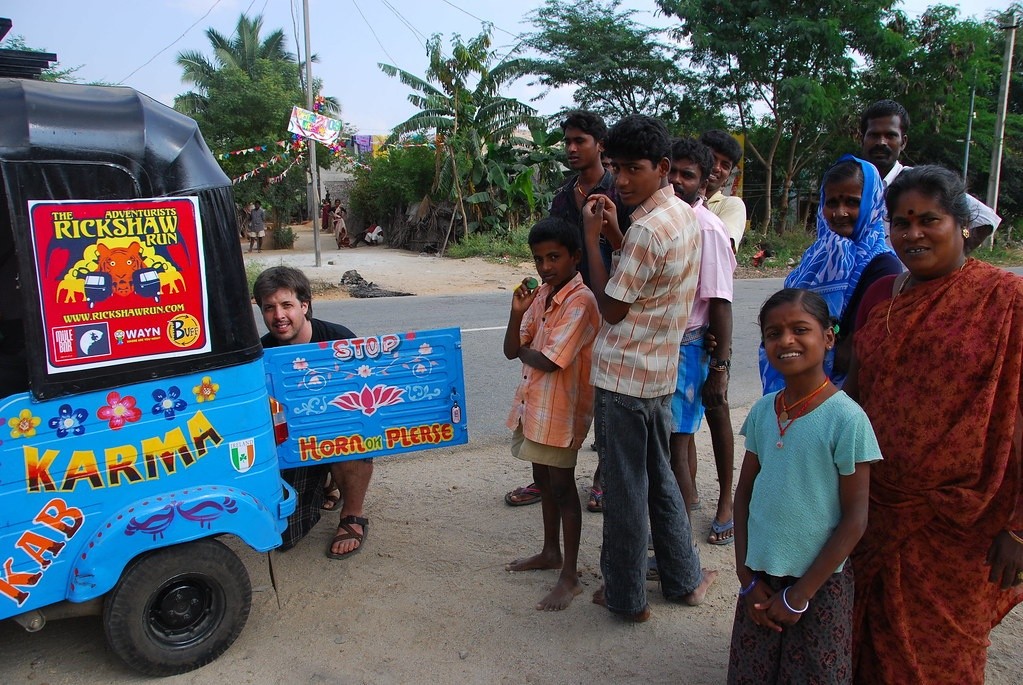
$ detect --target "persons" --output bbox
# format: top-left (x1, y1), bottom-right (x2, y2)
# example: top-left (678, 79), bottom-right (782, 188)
top-left (726, 99), bottom-right (1023, 685)
top-left (245, 199), bottom-right (267, 254)
top-left (504, 111), bottom-right (747, 623)
top-left (246, 265), bottom-right (374, 560)
top-left (321, 193), bottom-right (385, 250)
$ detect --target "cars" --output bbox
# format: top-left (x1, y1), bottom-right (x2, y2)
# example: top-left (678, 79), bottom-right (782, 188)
top-left (0, 83), bottom-right (474, 677)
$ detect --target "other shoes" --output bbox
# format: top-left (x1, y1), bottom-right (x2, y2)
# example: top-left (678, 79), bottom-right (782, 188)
top-left (648, 534), bottom-right (654, 548)
top-left (646, 554), bottom-right (658, 580)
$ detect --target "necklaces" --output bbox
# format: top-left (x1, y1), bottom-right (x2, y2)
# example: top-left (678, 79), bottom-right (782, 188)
top-left (775, 375), bottom-right (829, 423)
top-left (776, 375), bottom-right (830, 448)
top-left (576, 178), bottom-right (588, 197)
top-left (886, 258), bottom-right (968, 336)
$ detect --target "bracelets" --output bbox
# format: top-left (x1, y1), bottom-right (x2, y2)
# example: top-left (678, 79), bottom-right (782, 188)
top-left (783, 586), bottom-right (809, 614)
top-left (708, 357), bottom-right (730, 371)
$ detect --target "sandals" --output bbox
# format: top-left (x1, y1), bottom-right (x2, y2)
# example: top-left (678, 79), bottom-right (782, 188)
top-left (327, 515), bottom-right (369, 560)
top-left (321, 480), bottom-right (344, 511)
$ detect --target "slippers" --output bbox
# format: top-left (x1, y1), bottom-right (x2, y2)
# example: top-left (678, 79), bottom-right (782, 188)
top-left (588, 489), bottom-right (603, 511)
top-left (505, 482), bottom-right (541, 505)
top-left (707, 518), bottom-right (734, 545)
